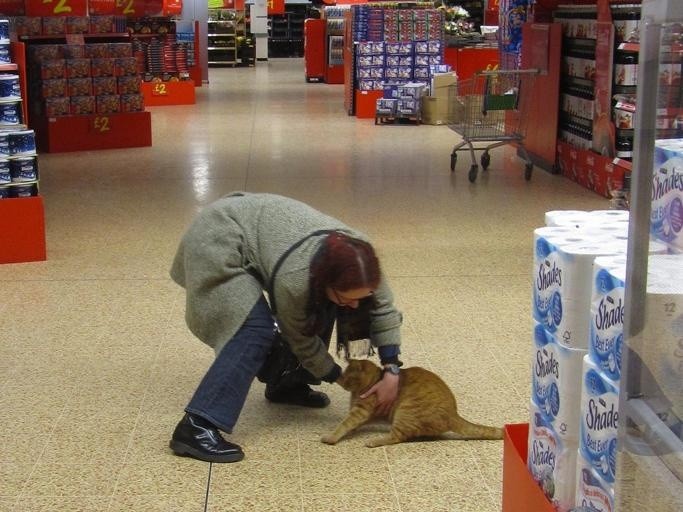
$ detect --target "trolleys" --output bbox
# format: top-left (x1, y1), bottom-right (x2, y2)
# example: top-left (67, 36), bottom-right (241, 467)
top-left (444, 68), bottom-right (547, 182)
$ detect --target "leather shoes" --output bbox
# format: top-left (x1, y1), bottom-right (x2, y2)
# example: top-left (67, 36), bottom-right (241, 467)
top-left (170, 414), bottom-right (243, 463)
top-left (266, 383), bottom-right (330, 407)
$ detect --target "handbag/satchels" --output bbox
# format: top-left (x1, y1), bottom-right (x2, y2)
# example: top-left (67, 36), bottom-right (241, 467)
top-left (258, 328), bottom-right (321, 385)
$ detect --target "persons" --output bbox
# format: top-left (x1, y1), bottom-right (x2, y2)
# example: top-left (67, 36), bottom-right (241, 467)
top-left (170, 191), bottom-right (403, 463)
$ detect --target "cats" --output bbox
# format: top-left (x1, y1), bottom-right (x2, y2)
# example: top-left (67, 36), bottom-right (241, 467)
top-left (321, 356), bottom-right (506, 448)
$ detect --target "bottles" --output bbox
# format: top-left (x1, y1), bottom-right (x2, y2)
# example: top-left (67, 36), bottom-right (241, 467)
top-left (327, 5), bottom-right (349, 65)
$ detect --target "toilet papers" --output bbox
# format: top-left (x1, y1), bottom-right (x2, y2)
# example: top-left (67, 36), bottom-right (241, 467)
top-left (524, 139), bottom-right (682, 511)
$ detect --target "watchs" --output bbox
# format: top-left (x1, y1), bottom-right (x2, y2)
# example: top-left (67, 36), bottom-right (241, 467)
top-left (383, 365), bottom-right (401, 376)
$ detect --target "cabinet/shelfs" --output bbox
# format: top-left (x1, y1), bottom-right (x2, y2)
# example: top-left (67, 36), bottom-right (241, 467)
top-left (323, 1), bottom-right (444, 118)
top-left (208, 1), bottom-right (304, 67)
top-left (497, 0), bottom-right (682, 198)
